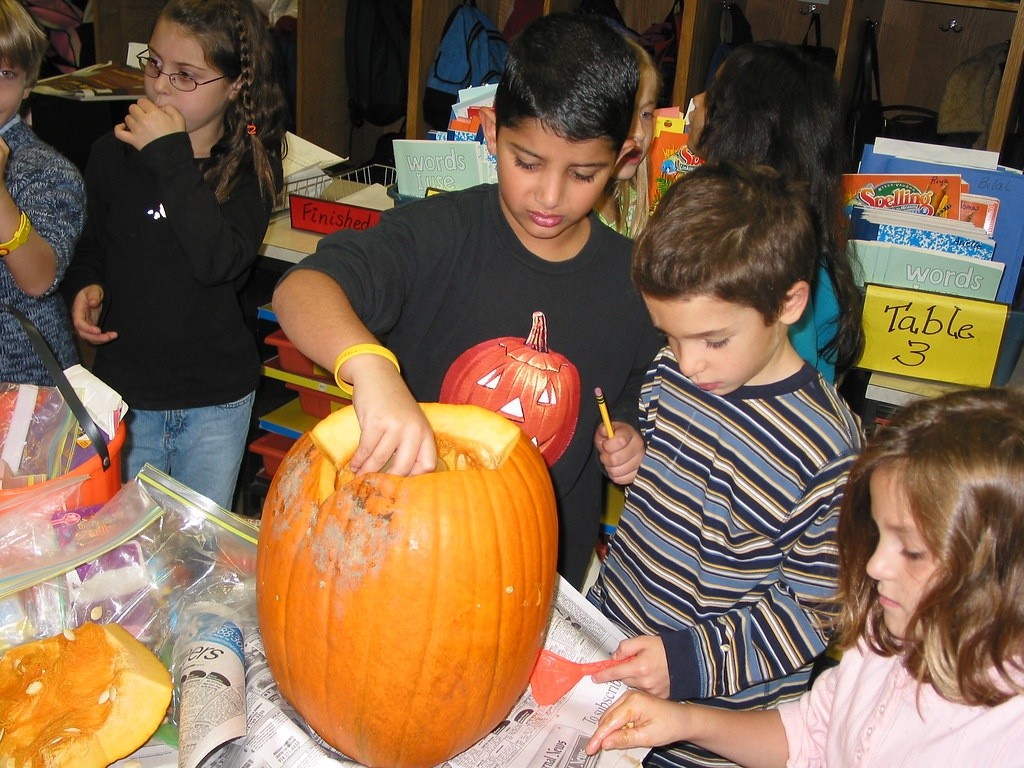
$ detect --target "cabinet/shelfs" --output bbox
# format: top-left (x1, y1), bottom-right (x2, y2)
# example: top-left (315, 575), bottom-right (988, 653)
top-left (248, 216), bottom-right (354, 520)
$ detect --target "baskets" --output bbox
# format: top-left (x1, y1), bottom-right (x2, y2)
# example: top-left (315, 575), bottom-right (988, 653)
top-left (268, 174), bottom-right (332, 225)
top-left (284, 163), bottom-right (398, 240)
top-left (0, 301), bottom-right (126, 563)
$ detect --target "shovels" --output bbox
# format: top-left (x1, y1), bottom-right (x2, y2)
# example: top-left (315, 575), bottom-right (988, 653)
top-left (530, 649), bottom-right (636, 706)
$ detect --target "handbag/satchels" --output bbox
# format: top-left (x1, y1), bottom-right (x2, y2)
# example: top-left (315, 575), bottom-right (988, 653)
top-left (845, 99), bottom-right (885, 161)
top-left (879, 104), bottom-right (941, 146)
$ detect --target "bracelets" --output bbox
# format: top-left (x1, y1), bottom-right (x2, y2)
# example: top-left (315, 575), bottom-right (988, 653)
top-left (334, 344), bottom-right (400, 396)
top-left (0, 206), bottom-right (32, 255)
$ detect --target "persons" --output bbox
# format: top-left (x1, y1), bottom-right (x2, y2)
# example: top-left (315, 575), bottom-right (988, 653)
top-left (585, 387), bottom-right (1024, 768)
top-left (688, 40), bottom-right (866, 386)
top-left (271, 12), bottom-right (668, 590)
top-left (582, 161), bottom-right (885, 768)
top-left (0, 0), bottom-right (291, 511)
top-left (592, 38), bottom-right (661, 240)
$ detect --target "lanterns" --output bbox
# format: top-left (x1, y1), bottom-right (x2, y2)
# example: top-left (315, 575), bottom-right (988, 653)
top-left (437, 310), bottom-right (580, 470)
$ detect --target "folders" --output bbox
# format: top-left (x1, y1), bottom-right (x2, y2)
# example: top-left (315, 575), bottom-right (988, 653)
top-left (858, 144), bottom-right (1023, 304)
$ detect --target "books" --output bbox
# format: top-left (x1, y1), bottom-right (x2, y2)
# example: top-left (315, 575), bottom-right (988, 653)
top-left (835, 138), bottom-right (1024, 437)
top-left (392, 82), bottom-right (499, 198)
top-left (648, 97), bottom-right (704, 212)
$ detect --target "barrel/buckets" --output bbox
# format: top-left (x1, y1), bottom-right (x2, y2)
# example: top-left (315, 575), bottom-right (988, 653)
top-left (1, 306), bottom-right (127, 523)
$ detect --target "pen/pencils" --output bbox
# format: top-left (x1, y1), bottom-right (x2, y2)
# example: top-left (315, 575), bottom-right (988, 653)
top-left (595, 388), bottom-right (614, 438)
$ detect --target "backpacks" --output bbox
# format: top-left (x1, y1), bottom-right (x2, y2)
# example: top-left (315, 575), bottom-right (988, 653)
top-left (792, 10), bottom-right (836, 78)
top-left (263, 15), bottom-right (298, 134)
top-left (422, 1), bottom-right (511, 131)
top-left (641, 0), bottom-right (684, 111)
top-left (704, 0), bottom-right (756, 99)
top-left (356, 119), bottom-right (406, 186)
top-left (343, 0), bottom-right (411, 126)
top-left (31, 0), bottom-right (96, 76)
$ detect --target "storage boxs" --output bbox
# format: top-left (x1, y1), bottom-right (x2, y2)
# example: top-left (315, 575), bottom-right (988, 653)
top-left (250, 431), bottom-right (296, 477)
top-left (264, 330), bottom-right (336, 381)
top-left (284, 383), bottom-right (353, 419)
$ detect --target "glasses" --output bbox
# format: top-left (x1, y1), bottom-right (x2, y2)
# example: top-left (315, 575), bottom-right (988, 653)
top-left (136, 49), bottom-right (228, 93)
